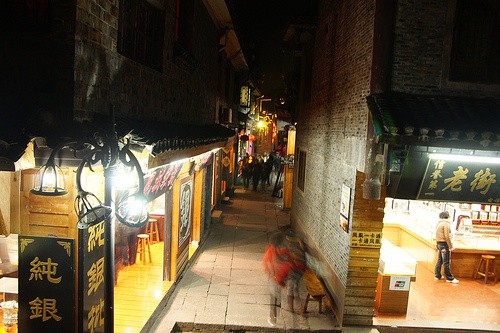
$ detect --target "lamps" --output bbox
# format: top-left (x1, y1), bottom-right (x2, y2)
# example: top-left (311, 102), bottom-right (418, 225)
top-left (239, 84), bottom-right (252, 108)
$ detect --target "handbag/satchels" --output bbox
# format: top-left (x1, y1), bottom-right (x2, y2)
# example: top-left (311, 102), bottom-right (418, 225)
top-left (432, 231), bottom-right (436, 240)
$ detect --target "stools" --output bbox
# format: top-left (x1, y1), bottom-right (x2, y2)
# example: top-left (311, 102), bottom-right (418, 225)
top-left (146, 219), bottom-right (159, 243)
top-left (134, 234), bottom-right (152, 264)
top-left (474, 255), bottom-right (497, 284)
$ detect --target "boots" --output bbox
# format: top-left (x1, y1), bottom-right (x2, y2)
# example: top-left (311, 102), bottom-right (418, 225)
top-left (296, 296), bottom-right (306, 317)
top-left (286, 294), bottom-right (295, 314)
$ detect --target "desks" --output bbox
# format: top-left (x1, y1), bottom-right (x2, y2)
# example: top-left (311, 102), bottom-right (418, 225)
top-left (0, 277), bottom-right (18, 302)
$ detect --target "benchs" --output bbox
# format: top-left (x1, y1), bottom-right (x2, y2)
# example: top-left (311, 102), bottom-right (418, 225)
top-left (301, 267), bottom-right (325, 314)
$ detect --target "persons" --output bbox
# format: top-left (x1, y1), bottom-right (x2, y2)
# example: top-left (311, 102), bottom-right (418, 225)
top-left (242, 155), bottom-right (273, 190)
top-left (119, 222), bottom-right (138, 265)
top-left (433, 212), bottom-right (458, 283)
top-left (264, 229), bottom-right (305, 323)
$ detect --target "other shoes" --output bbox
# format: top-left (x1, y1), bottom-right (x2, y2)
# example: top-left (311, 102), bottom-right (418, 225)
top-left (446, 278), bottom-right (459, 283)
top-left (268, 317), bottom-right (275, 327)
top-left (435, 276), bottom-right (444, 280)
